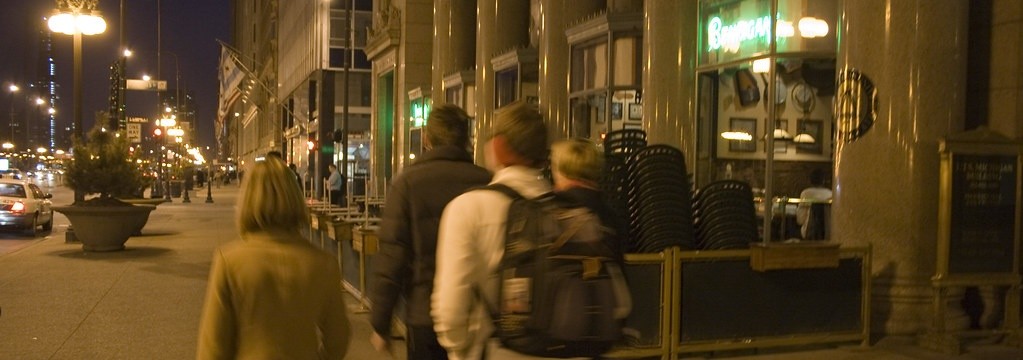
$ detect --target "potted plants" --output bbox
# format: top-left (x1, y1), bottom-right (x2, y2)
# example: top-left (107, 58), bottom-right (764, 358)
top-left (309, 210), bottom-right (379, 256)
top-left (48, 111), bottom-right (172, 253)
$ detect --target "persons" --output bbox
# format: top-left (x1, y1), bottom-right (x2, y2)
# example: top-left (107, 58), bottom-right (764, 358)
top-left (731, 160), bottom-right (754, 190)
top-left (368, 104), bottom-right (495, 360)
top-left (196, 167), bottom-right (243, 190)
top-left (267, 151), bottom-right (304, 198)
top-left (796, 188), bottom-right (832, 240)
top-left (324, 164), bottom-right (341, 205)
top-left (430, 100), bottom-right (626, 360)
top-left (196, 155), bottom-right (353, 360)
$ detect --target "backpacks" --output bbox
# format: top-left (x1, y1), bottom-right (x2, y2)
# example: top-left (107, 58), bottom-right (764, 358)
top-left (472, 184), bottom-right (622, 359)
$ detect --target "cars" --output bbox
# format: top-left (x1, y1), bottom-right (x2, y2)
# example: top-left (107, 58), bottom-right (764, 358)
top-left (0, 179), bottom-right (54, 236)
top-left (0, 168), bottom-right (57, 180)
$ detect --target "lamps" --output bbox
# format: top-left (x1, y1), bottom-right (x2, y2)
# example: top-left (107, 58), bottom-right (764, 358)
top-left (760, 64), bottom-right (815, 144)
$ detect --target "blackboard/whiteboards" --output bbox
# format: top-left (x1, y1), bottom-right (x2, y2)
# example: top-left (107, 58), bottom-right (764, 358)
top-left (940, 127), bottom-right (1023, 283)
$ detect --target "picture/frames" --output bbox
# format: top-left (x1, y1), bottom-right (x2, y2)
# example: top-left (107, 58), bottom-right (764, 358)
top-left (629, 103), bottom-right (642, 120)
top-left (728, 117), bottom-right (756, 153)
top-left (611, 102), bottom-right (623, 120)
top-left (796, 119), bottom-right (824, 156)
top-left (763, 117), bottom-right (788, 153)
top-left (596, 96), bottom-right (606, 123)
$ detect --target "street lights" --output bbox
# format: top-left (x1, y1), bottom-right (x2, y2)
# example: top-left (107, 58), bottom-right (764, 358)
top-left (155, 108), bottom-right (186, 199)
top-left (9, 83), bottom-right (57, 168)
top-left (48, 1), bottom-right (108, 244)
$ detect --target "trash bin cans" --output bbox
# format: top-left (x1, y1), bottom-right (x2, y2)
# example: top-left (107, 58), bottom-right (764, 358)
top-left (151, 180), bottom-right (163, 198)
top-left (171, 181), bottom-right (181, 199)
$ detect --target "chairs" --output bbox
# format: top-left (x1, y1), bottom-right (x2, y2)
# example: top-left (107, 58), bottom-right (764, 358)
top-left (598, 129), bottom-right (761, 343)
top-left (805, 202), bottom-right (832, 241)
